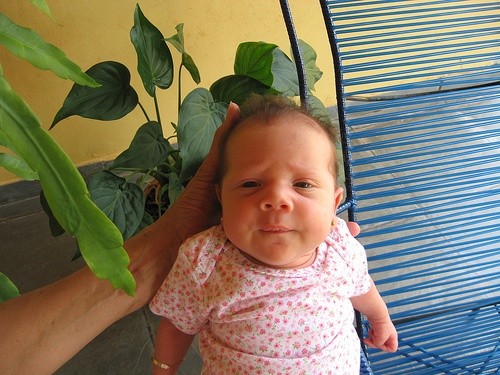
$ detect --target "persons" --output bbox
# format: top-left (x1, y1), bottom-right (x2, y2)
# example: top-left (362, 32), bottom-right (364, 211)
top-left (0, 98), bottom-right (361, 374)
top-left (148, 95), bottom-right (397, 374)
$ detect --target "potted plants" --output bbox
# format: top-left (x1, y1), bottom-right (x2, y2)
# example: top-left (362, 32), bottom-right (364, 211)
top-left (38, 3), bottom-right (326, 264)
